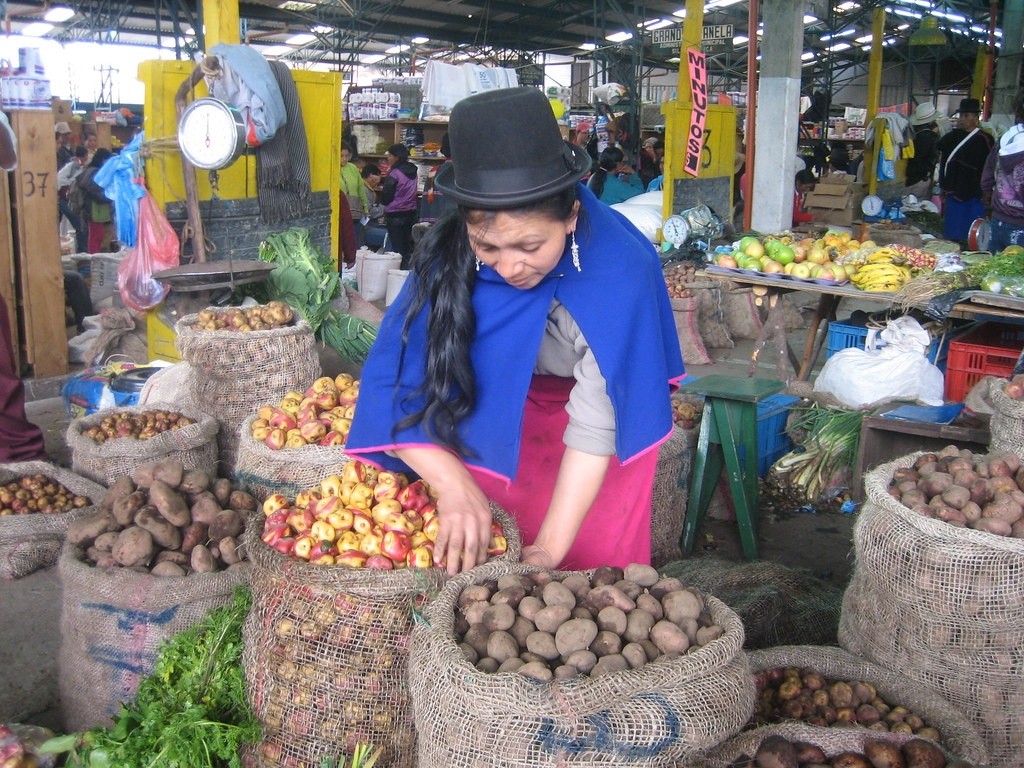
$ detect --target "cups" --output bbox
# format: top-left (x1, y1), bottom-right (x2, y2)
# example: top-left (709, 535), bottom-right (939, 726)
top-left (397, 109), bottom-right (411, 119)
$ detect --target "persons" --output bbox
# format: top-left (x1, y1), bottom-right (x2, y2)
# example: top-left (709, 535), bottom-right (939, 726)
top-left (793, 168), bottom-right (820, 227)
top-left (61, 271), bottom-right (93, 335)
top-left (906, 88), bottom-right (1024, 253)
top-left (574, 123), bottom-right (665, 205)
top-left (341, 87), bottom-right (687, 577)
top-left (828, 149), bottom-right (849, 175)
top-left (56, 122), bottom-right (116, 255)
top-left (339, 134), bottom-right (456, 274)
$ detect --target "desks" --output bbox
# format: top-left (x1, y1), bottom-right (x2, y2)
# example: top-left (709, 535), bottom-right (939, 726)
top-left (704, 266), bottom-right (1024, 380)
top-left (799, 137), bottom-right (865, 142)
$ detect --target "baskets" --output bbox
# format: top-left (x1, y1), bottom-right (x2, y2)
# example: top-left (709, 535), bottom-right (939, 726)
top-left (827, 307), bottom-right (977, 380)
top-left (945, 321), bottom-right (1021, 403)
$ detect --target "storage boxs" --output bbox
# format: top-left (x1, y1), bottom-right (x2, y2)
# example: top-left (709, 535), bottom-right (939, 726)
top-left (827, 311), bottom-right (977, 376)
top-left (738, 393), bottom-right (801, 477)
top-left (945, 320), bottom-right (1024, 402)
top-left (799, 116), bottom-right (866, 160)
top-left (802, 173), bottom-right (868, 226)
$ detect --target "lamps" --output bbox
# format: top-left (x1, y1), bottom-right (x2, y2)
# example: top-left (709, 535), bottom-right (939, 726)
top-left (908, 0), bottom-right (948, 46)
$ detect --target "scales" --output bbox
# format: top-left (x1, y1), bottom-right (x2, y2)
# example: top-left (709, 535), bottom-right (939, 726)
top-left (151, 76), bottom-right (279, 292)
top-left (862, 195), bottom-right (884, 222)
top-left (960, 217), bottom-right (993, 263)
top-left (662, 215), bottom-right (691, 251)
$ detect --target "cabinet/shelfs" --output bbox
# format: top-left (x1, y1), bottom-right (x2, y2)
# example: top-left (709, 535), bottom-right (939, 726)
top-left (349, 120), bottom-right (448, 195)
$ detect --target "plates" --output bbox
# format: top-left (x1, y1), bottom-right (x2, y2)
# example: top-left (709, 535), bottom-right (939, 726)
top-left (422, 116), bottom-right (450, 122)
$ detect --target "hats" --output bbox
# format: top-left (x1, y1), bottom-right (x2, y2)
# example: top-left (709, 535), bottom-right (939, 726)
top-left (956, 97), bottom-right (982, 114)
top-left (907, 102), bottom-right (942, 126)
top-left (433, 83), bottom-right (593, 206)
top-left (645, 137), bottom-right (658, 146)
top-left (76, 146), bottom-right (88, 158)
top-left (575, 122), bottom-right (590, 133)
top-left (55, 122), bottom-right (71, 134)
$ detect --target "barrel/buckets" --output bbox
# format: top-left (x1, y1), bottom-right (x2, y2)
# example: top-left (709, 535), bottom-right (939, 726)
top-left (108, 367), bottom-right (164, 408)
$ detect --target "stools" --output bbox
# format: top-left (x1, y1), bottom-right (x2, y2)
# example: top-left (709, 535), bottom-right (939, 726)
top-left (678, 374), bottom-right (786, 562)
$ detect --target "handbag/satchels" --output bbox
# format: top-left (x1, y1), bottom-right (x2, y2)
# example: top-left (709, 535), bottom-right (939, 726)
top-left (92, 200), bottom-right (111, 223)
top-left (118, 176), bottom-right (179, 310)
top-left (347, 195), bottom-right (363, 220)
